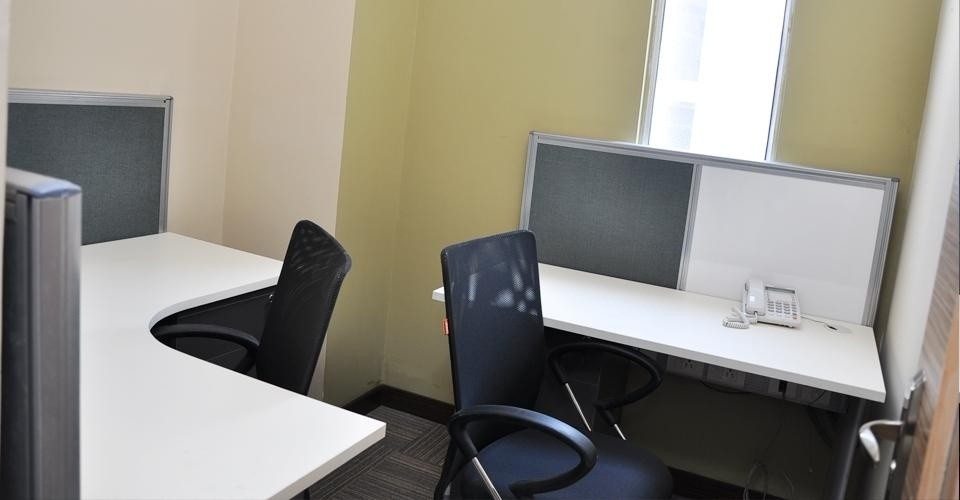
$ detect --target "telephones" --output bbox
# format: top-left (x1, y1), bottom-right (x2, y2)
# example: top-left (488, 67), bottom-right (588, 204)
top-left (743, 279), bottom-right (803, 328)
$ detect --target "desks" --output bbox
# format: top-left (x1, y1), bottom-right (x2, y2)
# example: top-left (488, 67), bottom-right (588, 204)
top-left (77, 231), bottom-right (387, 498)
top-left (431, 260), bottom-right (886, 500)
